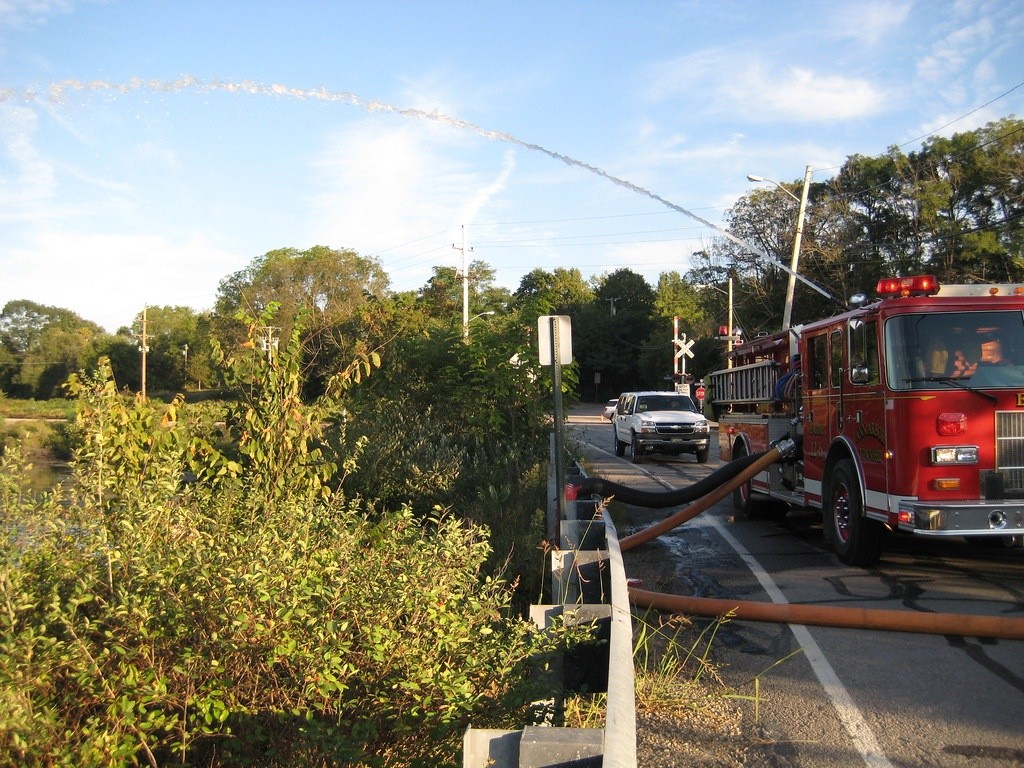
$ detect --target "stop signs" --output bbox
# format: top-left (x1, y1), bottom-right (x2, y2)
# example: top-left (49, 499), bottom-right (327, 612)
top-left (696, 387), bottom-right (706, 401)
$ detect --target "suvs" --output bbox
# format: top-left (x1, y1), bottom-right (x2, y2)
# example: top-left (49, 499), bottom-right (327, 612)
top-left (613, 391), bottom-right (711, 463)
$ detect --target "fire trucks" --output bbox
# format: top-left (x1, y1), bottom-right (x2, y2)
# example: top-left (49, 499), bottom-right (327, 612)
top-left (701, 273), bottom-right (1024, 565)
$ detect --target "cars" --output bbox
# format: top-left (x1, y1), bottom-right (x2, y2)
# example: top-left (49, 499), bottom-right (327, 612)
top-left (603, 398), bottom-right (619, 425)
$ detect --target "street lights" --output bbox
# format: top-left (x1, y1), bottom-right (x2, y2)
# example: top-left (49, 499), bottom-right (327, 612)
top-left (746, 164), bottom-right (815, 330)
